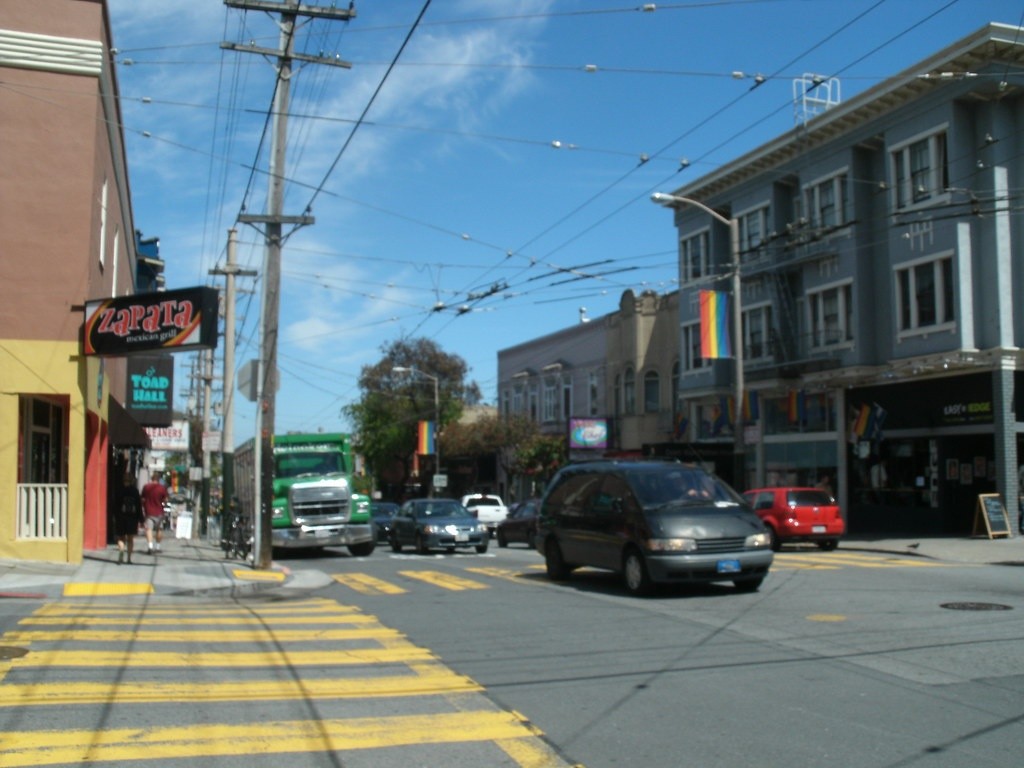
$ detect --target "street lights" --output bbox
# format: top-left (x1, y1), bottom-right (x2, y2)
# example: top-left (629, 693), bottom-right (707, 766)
top-left (392, 367), bottom-right (440, 500)
top-left (496, 500), bottom-right (542, 549)
top-left (651, 193), bottom-right (745, 493)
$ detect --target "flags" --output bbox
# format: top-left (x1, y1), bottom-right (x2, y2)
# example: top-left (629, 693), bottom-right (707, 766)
top-left (672, 286), bottom-right (879, 438)
top-left (408, 420), bottom-right (434, 478)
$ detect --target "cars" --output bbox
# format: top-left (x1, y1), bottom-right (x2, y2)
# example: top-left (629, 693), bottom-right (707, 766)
top-left (740, 487), bottom-right (844, 550)
top-left (373, 499), bottom-right (490, 554)
top-left (534, 458), bottom-right (774, 597)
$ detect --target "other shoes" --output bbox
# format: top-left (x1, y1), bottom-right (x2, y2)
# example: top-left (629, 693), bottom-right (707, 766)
top-left (155, 549), bottom-right (161, 553)
top-left (148, 549), bottom-right (153, 554)
top-left (126, 561), bottom-right (132, 564)
top-left (119, 551), bottom-right (124, 564)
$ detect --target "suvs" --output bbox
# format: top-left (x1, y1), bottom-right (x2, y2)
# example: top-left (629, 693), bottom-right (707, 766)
top-left (459, 493), bottom-right (510, 538)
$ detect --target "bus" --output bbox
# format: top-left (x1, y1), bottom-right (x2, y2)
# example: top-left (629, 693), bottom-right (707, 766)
top-left (234, 432), bottom-right (376, 558)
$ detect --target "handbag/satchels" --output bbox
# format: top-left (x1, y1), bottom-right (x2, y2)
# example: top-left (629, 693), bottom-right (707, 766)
top-left (119, 492), bottom-right (141, 521)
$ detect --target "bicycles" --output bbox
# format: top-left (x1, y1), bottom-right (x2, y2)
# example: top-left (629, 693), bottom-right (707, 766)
top-left (221, 506), bottom-right (249, 560)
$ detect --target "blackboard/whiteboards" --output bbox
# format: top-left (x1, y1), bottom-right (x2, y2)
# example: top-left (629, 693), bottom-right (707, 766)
top-left (972, 494), bottom-right (1011, 535)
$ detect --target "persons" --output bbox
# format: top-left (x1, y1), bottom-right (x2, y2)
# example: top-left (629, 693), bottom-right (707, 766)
top-left (111, 473), bottom-right (167, 565)
top-left (815, 474), bottom-right (831, 495)
